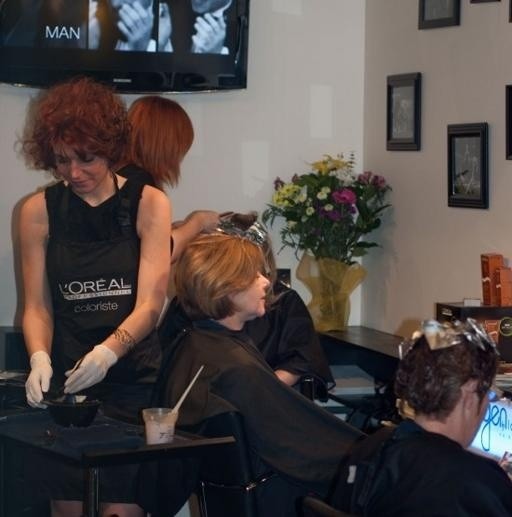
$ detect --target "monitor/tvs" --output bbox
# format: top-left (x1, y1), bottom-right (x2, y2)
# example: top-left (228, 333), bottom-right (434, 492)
top-left (0, 0), bottom-right (250, 95)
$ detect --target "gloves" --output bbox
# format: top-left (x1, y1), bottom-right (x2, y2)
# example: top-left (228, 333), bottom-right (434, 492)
top-left (24, 350), bottom-right (54, 410)
top-left (63, 344), bottom-right (118, 395)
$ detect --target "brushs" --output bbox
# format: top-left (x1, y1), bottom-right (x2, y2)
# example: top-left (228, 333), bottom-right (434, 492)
top-left (326, 311), bottom-right (512, 517)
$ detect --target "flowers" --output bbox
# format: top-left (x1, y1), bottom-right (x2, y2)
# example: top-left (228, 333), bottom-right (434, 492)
top-left (261, 151), bottom-right (391, 329)
top-left (418, 0), bottom-right (460, 29)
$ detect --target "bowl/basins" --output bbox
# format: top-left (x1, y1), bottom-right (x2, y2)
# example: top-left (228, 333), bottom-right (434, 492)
top-left (43, 393), bottom-right (105, 427)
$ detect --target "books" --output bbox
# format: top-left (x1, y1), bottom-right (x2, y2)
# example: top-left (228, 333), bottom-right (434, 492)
top-left (493, 370), bottom-right (512, 396)
top-left (323, 363), bottom-right (386, 397)
top-left (499, 451), bottom-right (511, 480)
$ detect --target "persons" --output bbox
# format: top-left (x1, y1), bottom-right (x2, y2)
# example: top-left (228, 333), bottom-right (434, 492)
top-left (159, 0), bottom-right (241, 55)
top-left (17, 75), bottom-right (172, 517)
top-left (161, 213), bottom-right (336, 402)
top-left (135, 233), bottom-right (368, 517)
top-left (88, 1), bottom-right (158, 53)
top-left (124, 96), bottom-right (224, 265)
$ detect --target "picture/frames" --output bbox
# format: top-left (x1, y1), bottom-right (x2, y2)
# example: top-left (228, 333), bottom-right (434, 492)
top-left (387, 72), bottom-right (421, 151)
top-left (446, 123), bottom-right (489, 209)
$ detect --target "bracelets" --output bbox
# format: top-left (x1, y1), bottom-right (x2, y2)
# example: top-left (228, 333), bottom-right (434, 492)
top-left (113, 327), bottom-right (135, 352)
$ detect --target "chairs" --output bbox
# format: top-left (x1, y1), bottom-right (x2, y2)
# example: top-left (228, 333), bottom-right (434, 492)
top-left (294, 496), bottom-right (350, 517)
top-left (167, 386), bottom-right (279, 517)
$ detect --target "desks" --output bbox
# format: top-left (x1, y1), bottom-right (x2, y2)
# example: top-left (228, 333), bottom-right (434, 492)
top-left (325, 323), bottom-right (404, 396)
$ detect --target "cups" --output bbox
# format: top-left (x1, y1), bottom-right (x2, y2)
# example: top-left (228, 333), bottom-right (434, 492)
top-left (142, 406), bottom-right (177, 443)
